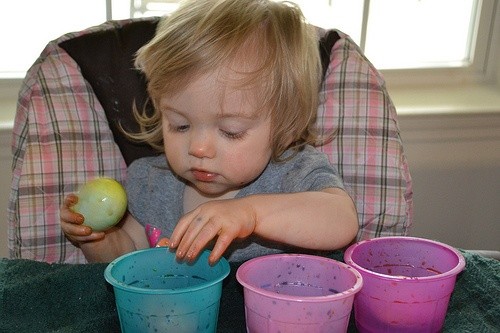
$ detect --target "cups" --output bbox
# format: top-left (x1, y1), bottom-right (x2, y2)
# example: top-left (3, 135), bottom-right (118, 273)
top-left (103, 247), bottom-right (231, 333)
top-left (344, 236), bottom-right (466, 332)
top-left (236, 253), bottom-right (364, 333)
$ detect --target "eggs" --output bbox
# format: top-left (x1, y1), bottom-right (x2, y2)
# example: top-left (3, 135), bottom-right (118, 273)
top-left (69, 178), bottom-right (128, 233)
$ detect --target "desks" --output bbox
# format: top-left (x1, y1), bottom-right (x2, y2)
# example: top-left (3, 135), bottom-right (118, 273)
top-left (0, 252), bottom-right (500, 333)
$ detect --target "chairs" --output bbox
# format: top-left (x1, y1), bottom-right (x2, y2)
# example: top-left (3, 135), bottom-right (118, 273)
top-left (8, 15), bottom-right (421, 243)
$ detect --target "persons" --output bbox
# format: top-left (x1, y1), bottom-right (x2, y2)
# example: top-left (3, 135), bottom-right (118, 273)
top-left (60, 1), bottom-right (360, 264)
